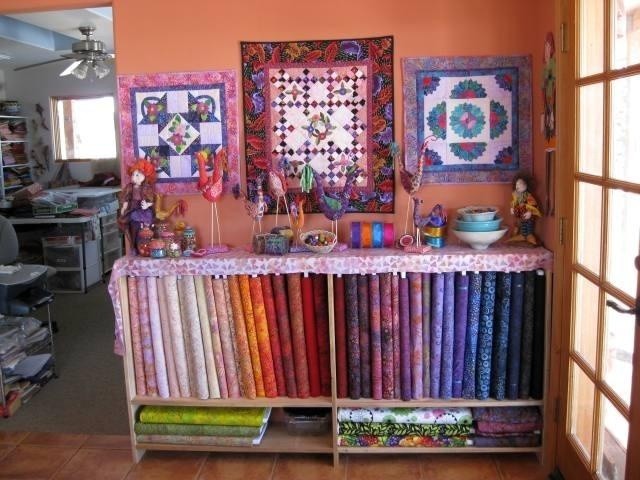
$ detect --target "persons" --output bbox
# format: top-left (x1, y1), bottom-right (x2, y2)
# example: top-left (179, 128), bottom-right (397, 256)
top-left (539, 32), bottom-right (556, 144)
top-left (119, 158), bottom-right (157, 256)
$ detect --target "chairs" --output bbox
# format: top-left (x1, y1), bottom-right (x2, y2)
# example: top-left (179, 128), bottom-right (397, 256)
top-left (0, 214), bottom-right (61, 337)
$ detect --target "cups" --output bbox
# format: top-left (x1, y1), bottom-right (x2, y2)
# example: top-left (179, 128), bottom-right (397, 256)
top-left (400, 234), bottom-right (414, 247)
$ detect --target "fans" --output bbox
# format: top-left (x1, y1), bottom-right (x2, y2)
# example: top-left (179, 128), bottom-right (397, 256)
top-left (12, 24), bottom-right (116, 77)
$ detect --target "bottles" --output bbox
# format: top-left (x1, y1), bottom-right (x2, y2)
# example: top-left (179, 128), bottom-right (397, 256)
top-left (252, 228), bottom-right (295, 256)
top-left (137, 222), bottom-right (196, 257)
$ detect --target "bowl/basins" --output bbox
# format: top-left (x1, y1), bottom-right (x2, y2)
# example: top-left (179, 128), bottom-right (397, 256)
top-left (452, 206), bottom-right (509, 251)
top-left (1, 101), bottom-right (21, 116)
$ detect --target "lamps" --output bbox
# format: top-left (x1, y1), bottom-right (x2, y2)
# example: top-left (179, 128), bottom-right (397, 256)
top-left (71, 57), bottom-right (112, 80)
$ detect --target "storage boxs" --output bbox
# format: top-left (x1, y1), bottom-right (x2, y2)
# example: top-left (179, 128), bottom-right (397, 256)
top-left (281, 410), bottom-right (331, 437)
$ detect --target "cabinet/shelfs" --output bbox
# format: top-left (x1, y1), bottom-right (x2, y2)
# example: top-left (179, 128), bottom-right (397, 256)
top-left (0, 115), bottom-right (37, 198)
top-left (0, 303), bottom-right (60, 419)
top-left (108, 245), bottom-right (336, 468)
top-left (6, 184), bottom-right (119, 295)
top-left (330, 240), bottom-right (553, 467)
